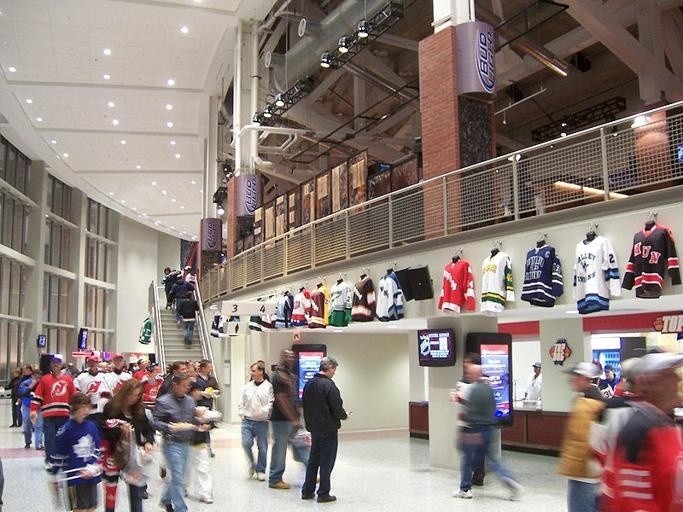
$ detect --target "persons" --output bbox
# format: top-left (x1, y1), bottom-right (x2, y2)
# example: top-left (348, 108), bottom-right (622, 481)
top-left (458, 353), bottom-right (488, 486)
top-left (275, 287), bottom-right (311, 329)
top-left (269, 348), bottom-right (320, 490)
top-left (620, 221), bottom-right (682, 300)
top-left (520, 240), bottom-right (564, 307)
top-left (523, 362), bottom-right (543, 401)
top-left (248, 360), bottom-right (270, 381)
top-left (480, 249), bottom-right (515, 318)
top-left (308, 278), bottom-right (353, 329)
top-left (248, 294), bottom-right (278, 332)
top-left (137, 317), bottom-right (153, 345)
top-left (349, 267), bottom-right (406, 323)
top-left (556, 346), bottom-right (683, 512)
top-left (438, 256), bottom-right (475, 315)
top-left (448, 363), bottom-right (527, 502)
top-left (241, 360), bottom-right (276, 482)
top-left (572, 231), bottom-right (621, 315)
top-left (160, 266), bottom-right (200, 348)
top-left (0, 352), bottom-right (222, 511)
top-left (302, 355), bottom-right (352, 502)
top-left (211, 313), bottom-right (240, 338)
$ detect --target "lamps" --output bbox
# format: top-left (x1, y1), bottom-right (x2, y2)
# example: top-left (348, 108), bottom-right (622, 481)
top-left (320, 17), bottom-right (372, 69)
top-left (262, 91), bottom-right (287, 117)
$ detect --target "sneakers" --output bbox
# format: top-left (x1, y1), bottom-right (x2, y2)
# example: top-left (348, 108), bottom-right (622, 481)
top-left (248, 464), bottom-right (257, 479)
top-left (452, 489), bottom-right (472, 498)
top-left (271, 481), bottom-right (290, 489)
top-left (257, 472), bottom-right (266, 481)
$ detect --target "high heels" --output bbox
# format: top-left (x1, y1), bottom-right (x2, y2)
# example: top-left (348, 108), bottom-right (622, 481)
top-left (200, 498), bottom-right (213, 503)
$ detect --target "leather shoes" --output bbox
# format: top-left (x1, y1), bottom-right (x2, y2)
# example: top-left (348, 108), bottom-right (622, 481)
top-left (142, 490), bottom-right (148, 498)
top-left (317, 496), bottom-right (337, 502)
top-left (471, 479), bottom-right (483, 485)
top-left (166, 503), bottom-right (174, 512)
top-left (302, 493), bottom-right (316, 499)
top-left (10, 424), bottom-right (45, 450)
top-left (161, 468), bottom-right (166, 478)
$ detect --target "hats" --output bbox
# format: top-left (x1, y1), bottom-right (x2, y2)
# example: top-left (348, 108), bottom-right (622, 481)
top-left (49, 354), bottom-right (62, 364)
top-left (603, 365), bottom-right (613, 372)
top-left (70, 393), bottom-right (94, 412)
top-left (563, 362), bottom-right (604, 378)
top-left (532, 362), bottom-right (541, 368)
top-left (87, 354), bottom-right (159, 372)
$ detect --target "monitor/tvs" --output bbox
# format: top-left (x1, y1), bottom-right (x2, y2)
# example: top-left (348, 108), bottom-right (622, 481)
top-left (78, 327), bottom-right (88, 350)
top-left (37, 334), bottom-right (46, 348)
top-left (417, 329), bottom-right (453, 366)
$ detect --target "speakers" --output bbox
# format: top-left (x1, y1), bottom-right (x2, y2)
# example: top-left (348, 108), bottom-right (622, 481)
top-left (569, 52), bottom-right (591, 73)
top-left (505, 83), bottom-right (524, 103)
